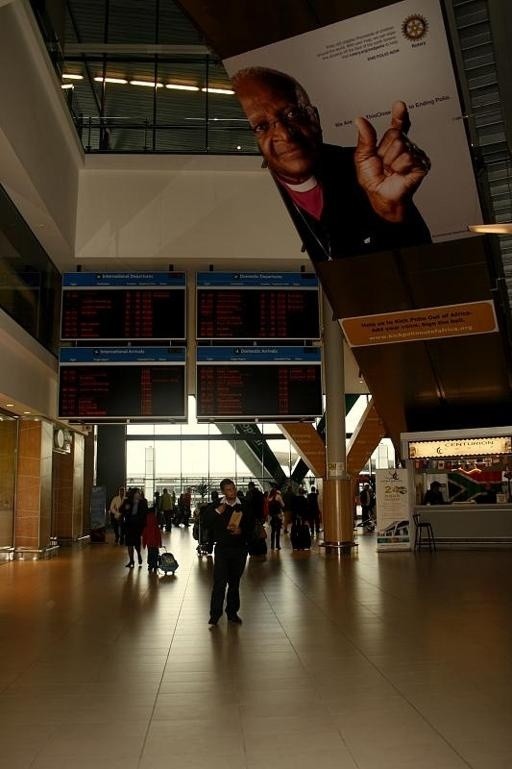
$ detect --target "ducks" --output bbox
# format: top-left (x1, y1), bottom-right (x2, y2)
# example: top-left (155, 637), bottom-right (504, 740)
top-left (290, 518), bottom-right (311, 550)
top-left (158, 546), bottom-right (179, 575)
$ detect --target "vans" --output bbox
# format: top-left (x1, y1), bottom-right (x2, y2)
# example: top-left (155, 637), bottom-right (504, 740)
top-left (138, 559), bottom-right (142, 564)
top-left (125, 560), bottom-right (134, 566)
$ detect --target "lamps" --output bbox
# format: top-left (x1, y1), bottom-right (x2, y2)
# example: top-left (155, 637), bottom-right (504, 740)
top-left (411, 513), bottom-right (436, 554)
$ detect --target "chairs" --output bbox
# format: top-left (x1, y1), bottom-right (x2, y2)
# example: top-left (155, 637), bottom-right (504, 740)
top-left (276, 546), bottom-right (281, 550)
top-left (114, 536), bottom-right (120, 542)
top-left (159, 524), bottom-right (191, 532)
top-left (121, 536), bottom-right (124, 543)
top-left (271, 547), bottom-right (274, 550)
top-left (225, 607), bottom-right (241, 624)
top-left (209, 611), bottom-right (222, 624)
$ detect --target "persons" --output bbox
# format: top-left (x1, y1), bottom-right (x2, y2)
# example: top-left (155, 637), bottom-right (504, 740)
top-left (230, 59), bottom-right (432, 257)
top-left (207, 479), bottom-right (253, 625)
